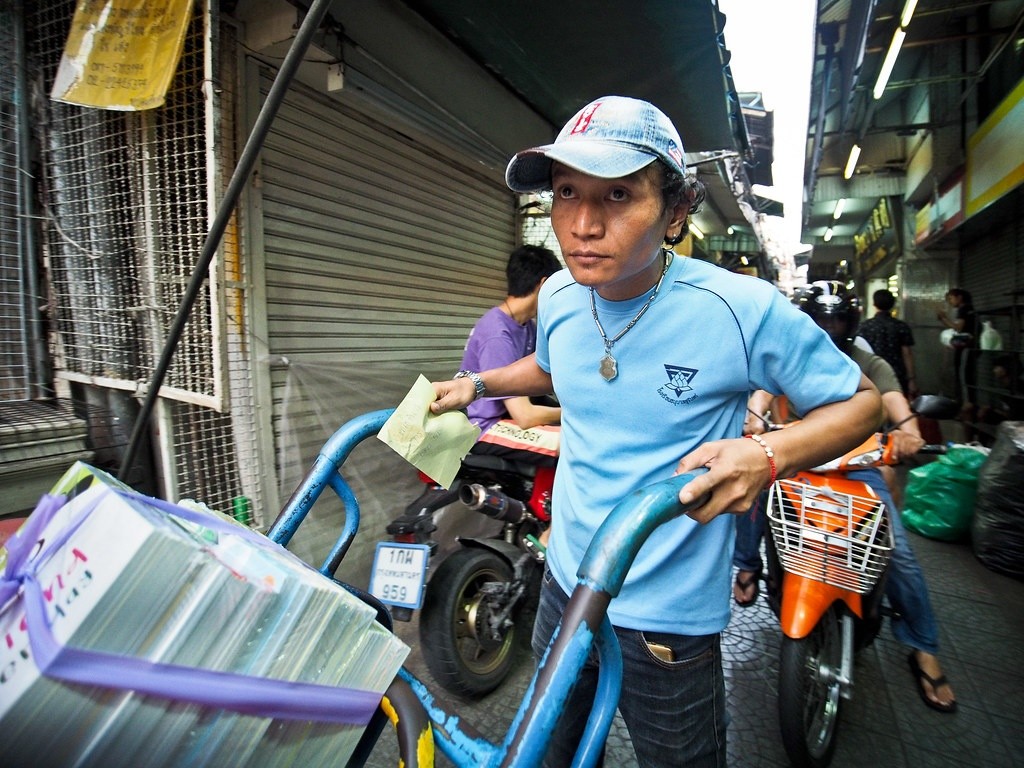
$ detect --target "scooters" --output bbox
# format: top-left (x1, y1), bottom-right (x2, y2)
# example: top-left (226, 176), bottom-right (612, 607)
top-left (367, 453), bottom-right (560, 702)
top-left (726, 425), bottom-right (947, 768)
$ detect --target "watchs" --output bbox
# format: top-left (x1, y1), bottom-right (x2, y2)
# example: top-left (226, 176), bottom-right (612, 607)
top-left (453, 370), bottom-right (486, 401)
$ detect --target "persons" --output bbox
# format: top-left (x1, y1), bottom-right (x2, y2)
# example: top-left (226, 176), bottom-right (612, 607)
top-left (936, 287), bottom-right (981, 410)
top-left (428, 95), bottom-right (883, 768)
top-left (733, 280), bottom-right (957, 716)
top-left (858, 288), bottom-right (916, 400)
top-left (461, 247), bottom-right (561, 563)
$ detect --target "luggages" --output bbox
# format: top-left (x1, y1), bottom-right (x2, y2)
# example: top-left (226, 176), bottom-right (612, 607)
top-left (906, 391), bottom-right (943, 445)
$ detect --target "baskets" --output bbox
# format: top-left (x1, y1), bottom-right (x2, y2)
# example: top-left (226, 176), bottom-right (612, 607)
top-left (766, 477), bottom-right (895, 595)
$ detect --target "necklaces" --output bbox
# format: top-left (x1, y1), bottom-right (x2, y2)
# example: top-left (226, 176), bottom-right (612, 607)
top-left (589, 247), bottom-right (669, 383)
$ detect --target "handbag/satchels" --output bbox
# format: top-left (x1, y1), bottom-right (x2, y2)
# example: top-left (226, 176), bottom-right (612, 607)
top-left (950, 326), bottom-right (970, 347)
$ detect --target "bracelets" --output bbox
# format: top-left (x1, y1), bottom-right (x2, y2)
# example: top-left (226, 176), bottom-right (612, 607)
top-left (745, 435), bottom-right (776, 489)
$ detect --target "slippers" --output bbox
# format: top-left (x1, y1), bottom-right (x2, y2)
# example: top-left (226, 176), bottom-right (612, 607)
top-left (733, 557), bottom-right (763, 607)
top-left (907, 647), bottom-right (956, 711)
top-left (527, 533), bottom-right (546, 554)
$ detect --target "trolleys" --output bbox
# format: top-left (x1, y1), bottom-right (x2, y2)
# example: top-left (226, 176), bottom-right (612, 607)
top-left (267, 406), bottom-right (718, 768)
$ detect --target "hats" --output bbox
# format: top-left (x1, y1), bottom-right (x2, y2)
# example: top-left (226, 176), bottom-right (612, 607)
top-left (505, 94), bottom-right (688, 196)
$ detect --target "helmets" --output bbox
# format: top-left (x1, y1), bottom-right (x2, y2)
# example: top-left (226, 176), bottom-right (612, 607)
top-left (798, 278), bottom-right (860, 353)
